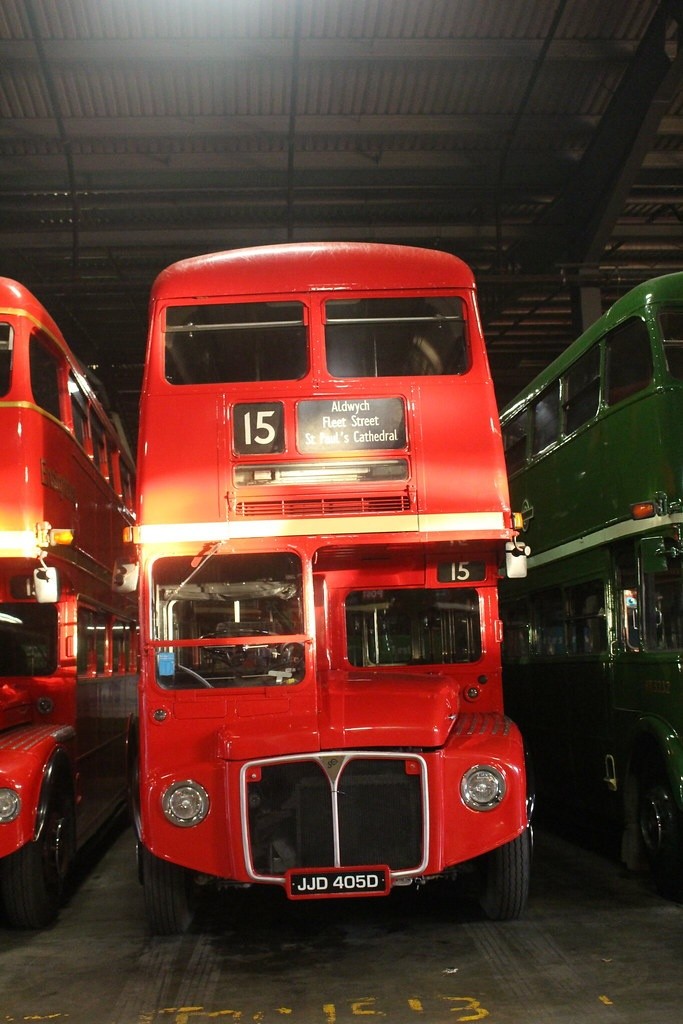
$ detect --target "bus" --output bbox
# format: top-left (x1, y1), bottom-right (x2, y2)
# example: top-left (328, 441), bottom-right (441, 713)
top-left (123, 244), bottom-right (535, 943)
top-left (488, 273), bottom-right (683, 894)
top-left (0, 271), bottom-right (144, 934)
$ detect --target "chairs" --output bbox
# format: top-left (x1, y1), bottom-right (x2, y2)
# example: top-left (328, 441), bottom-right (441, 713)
top-left (198, 615), bottom-right (682, 678)
